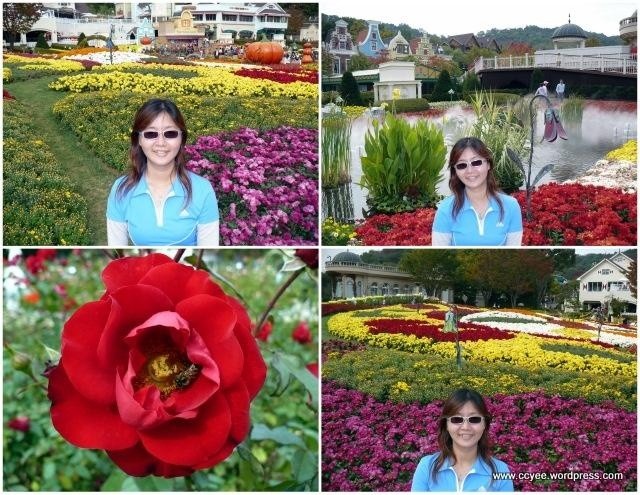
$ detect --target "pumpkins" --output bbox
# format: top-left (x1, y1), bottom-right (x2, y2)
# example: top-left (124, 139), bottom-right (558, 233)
top-left (302, 39), bottom-right (313, 64)
top-left (141, 33), bottom-right (152, 45)
top-left (246, 32), bottom-right (285, 64)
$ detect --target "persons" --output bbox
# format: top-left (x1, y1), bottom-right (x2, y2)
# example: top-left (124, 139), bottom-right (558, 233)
top-left (555, 78), bottom-right (566, 102)
top-left (534, 82), bottom-right (547, 96)
top-left (430, 136), bottom-right (525, 245)
top-left (214, 36), bottom-right (299, 64)
top-left (407, 387), bottom-right (516, 492)
top-left (542, 80), bottom-right (549, 95)
top-left (105, 98), bottom-right (221, 246)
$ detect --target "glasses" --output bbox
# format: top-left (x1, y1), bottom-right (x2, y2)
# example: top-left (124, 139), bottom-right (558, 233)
top-left (453, 159), bottom-right (483, 169)
top-left (450, 416), bottom-right (482, 423)
top-left (139, 130), bottom-right (183, 139)
